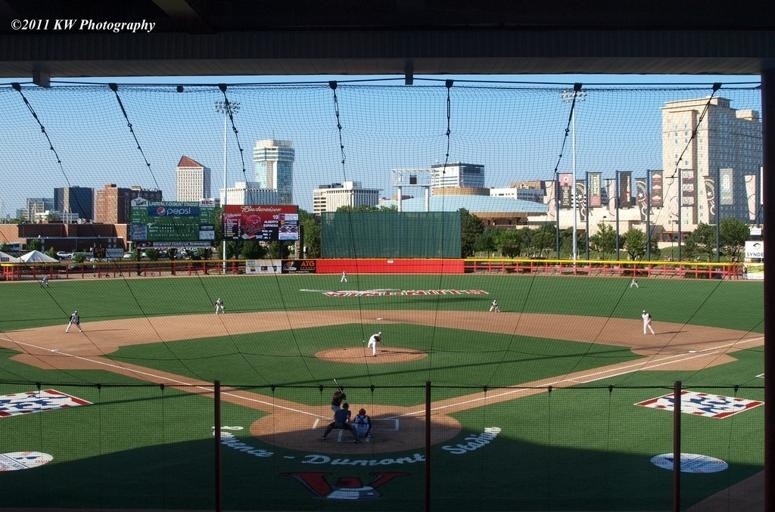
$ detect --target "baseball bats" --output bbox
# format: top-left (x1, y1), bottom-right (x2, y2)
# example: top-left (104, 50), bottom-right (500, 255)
top-left (333, 378), bottom-right (343, 395)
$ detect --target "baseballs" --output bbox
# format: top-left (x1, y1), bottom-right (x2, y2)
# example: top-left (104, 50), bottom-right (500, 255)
top-left (362, 340), bottom-right (365, 342)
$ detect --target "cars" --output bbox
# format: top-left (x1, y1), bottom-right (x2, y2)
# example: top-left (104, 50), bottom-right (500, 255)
top-left (55, 248), bottom-right (201, 259)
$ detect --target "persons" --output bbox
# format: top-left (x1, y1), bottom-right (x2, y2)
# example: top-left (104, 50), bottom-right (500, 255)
top-left (368, 331), bottom-right (382, 357)
top-left (640, 309), bottom-right (654, 334)
top-left (341, 271), bottom-right (347, 283)
top-left (40, 272), bottom-right (49, 288)
top-left (354, 408), bottom-right (371, 442)
top-left (215, 298), bottom-right (225, 314)
top-left (66, 309), bottom-right (83, 332)
top-left (331, 386), bottom-right (347, 412)
top-left (630, 278), bottom-right (639, 288)
top-left (322, 402), bottom-right (362, 443)
top-left (490, 299), bottom-right (501, 313)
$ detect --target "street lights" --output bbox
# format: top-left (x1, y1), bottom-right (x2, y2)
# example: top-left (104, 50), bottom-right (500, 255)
top-left (559, 85), bottom-right (588, 276)
top-left (603, 215), bottom-right (607, 261)
top-left (214, 98), bottom-right (242, 275)
